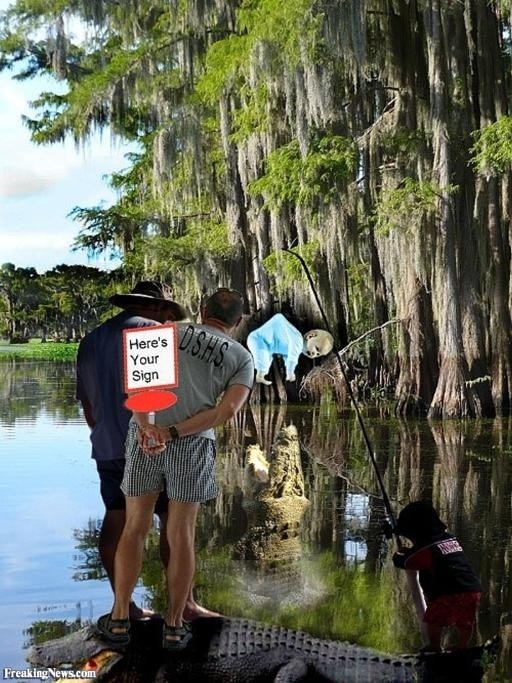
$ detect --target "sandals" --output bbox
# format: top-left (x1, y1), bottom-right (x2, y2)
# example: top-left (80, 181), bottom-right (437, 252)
top-left (96, 613), bottom-right (131, 643)
top-left (162, 620), bottom-right (193, 651)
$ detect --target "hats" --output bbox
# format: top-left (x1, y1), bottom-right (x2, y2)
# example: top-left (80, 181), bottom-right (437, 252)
top-left (391, 500), bottom-right (448, 536)
top-left (108, 280), bottom-right (186, 321)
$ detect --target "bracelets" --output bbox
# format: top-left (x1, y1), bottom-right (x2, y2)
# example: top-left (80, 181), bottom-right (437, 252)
top-left (168, 425), bottom-right (179, 441)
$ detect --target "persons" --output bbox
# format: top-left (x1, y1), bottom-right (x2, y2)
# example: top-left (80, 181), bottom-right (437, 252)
top-left (97, 289), bottom-right (256, 650)
top-left (247, 313), bottom-right (335, 386)
top-left (74, 280), bottom-right (225, 622)
top-left (391, 498), bottom-right (482, 652)
